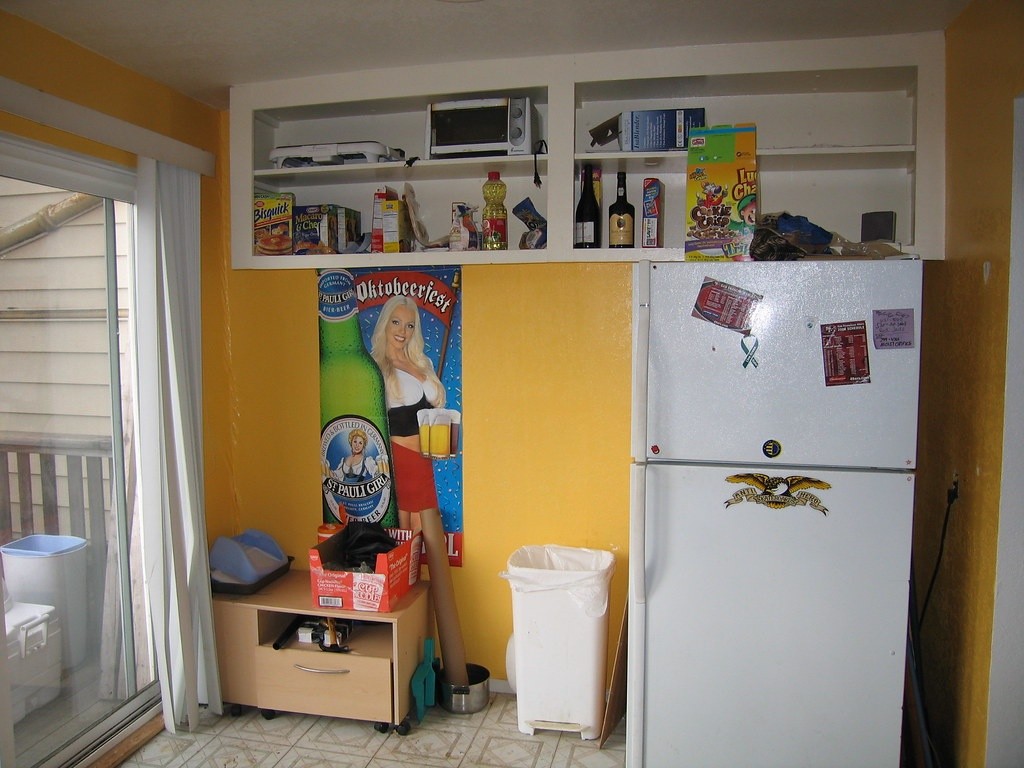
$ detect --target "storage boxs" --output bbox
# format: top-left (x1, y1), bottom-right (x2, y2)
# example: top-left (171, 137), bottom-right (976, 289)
top-left (588, 108), bottom-right (762, 261)
top-left (249, 182), bottom-right (416, 254)
top-left (4, 599), bottom-right (64, 727)
top-left (309, 523), bottom-right (423, 614)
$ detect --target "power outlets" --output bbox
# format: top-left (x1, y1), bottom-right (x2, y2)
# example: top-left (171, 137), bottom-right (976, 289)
top-left (952, 466), bottom-right (961, 505)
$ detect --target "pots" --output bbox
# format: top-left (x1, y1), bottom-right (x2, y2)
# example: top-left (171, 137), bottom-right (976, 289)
top-left (436, 663), bottom-right (491, 714)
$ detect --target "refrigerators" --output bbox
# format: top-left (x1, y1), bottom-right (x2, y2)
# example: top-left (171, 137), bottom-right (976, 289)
top-left (624, 256), bottom-right (923, 768)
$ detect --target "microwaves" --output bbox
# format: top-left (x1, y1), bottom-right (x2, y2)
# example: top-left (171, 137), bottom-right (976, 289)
top-left (423, 96), bottom-right (538, 160)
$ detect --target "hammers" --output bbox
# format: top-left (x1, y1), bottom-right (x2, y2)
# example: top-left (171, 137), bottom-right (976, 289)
top-left (319, 617), bottom-right (349, 653)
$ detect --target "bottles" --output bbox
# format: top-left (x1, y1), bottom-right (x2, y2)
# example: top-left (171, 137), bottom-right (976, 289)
top-left (317, 270), bottom-right (400, 530)
top-left (575, 164), bottom-right (602, 248)
top-left (482, 172), bottom-right (509, 250)
top-left (608, 171), bottom-right (635, 248)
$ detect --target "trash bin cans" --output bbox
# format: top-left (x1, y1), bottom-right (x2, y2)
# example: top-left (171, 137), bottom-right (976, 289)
top-left (505, 544), bottom-right (616, 740)
top-left (0, 534), bottom-right (89, 670)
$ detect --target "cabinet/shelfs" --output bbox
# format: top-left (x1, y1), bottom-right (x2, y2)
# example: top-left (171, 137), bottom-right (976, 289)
top-left (228, 35), bottom-right (947, 262)
top-left (207, 568), bottom-right (440, 736)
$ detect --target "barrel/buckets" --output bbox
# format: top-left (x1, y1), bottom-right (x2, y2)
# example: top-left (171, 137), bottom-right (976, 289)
top-left (0, 533), bottom-right (87, 670)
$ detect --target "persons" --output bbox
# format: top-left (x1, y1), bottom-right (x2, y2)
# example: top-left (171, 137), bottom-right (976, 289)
top-left (370, 295), bottom-right (446, 530)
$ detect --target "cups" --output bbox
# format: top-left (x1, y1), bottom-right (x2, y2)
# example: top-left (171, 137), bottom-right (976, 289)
top-left (417, 408), bottom-right (461, 459)
